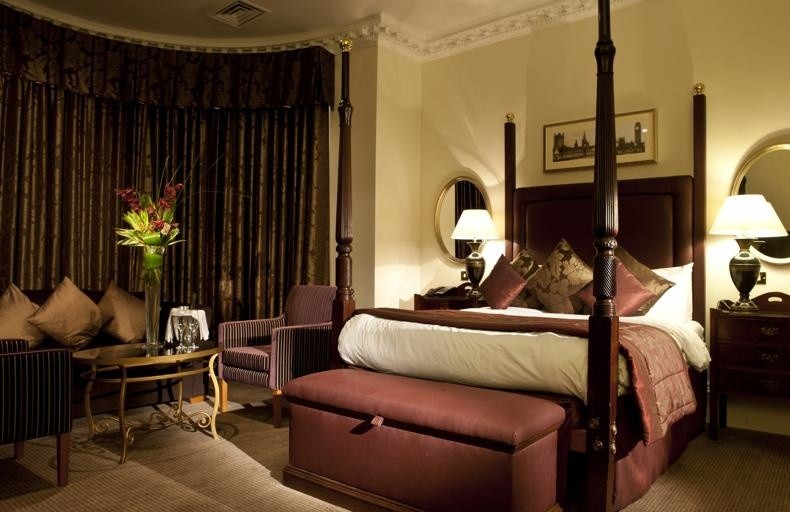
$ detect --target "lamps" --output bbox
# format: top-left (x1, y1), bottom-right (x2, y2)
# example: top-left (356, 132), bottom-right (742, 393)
top-left (711, 190), bottom-right (786, 312)
top-left (447, 208), bottom-right (503, 298)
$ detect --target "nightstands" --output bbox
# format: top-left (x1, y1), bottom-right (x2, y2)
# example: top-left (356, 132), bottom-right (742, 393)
top-left (414, 281), bottom-right (473, 311)
top-left (705, 290), bottom-right (790, 444)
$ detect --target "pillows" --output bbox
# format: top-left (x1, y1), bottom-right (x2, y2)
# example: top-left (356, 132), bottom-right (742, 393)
top-left (25, 278), bottom-right (114, 349)
top-left (0, 284), bottom-right (50, 349)
top-left (96, 278), bottom-right (161, 345)
top-left (478, 240), bottom-right (692, 324)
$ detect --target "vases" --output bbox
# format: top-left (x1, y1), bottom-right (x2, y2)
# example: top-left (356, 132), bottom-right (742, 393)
top-left (140, 244), bottom-right (167, 351)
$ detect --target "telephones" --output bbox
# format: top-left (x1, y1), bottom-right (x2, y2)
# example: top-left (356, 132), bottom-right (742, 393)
top-left (427, 287), bottom-right (456, 296)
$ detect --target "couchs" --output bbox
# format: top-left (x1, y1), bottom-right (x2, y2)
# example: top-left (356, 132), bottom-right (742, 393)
top-left (5, 288), bottom-right (213, 419)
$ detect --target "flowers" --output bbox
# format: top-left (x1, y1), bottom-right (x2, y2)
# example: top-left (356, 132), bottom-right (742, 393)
top-left (106, 151), bottom-right (249, 344)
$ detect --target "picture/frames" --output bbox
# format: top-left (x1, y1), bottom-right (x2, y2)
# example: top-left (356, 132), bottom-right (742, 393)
top-left (542, 108), bottom-right (659, 173)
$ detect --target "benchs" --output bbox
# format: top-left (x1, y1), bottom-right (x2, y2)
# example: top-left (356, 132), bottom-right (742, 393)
top-left (277, 365), bottom-right (567, 511)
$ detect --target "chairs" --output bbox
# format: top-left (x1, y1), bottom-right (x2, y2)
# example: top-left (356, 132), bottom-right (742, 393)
top-left (0, 337), bottom-right (75, 486)
top-left (216, 283), bottom-right (346, 428)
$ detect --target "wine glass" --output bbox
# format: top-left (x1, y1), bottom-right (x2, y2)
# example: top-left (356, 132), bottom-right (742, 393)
top-left (173, 306), bottom-right (200, 350)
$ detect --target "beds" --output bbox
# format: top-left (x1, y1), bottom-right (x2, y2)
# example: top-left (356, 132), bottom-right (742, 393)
top-left (333, 0), bottom-right (714, 512)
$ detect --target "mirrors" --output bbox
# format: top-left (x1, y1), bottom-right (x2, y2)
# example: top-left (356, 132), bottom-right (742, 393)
top-left (435, 176), bottom-right (494, 265)
top-left (727, 139), bottom-right (790, 266)
